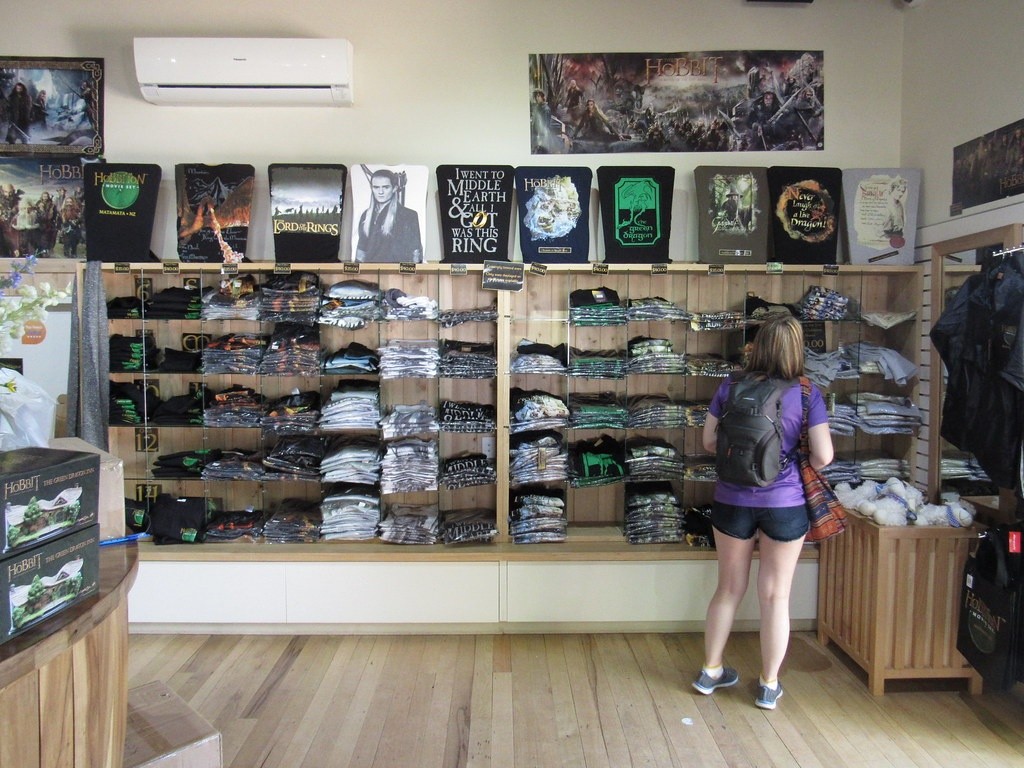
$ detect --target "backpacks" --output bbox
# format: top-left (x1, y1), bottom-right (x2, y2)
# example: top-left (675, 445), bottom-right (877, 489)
top-left (716, 370), bottom-right (803, 488)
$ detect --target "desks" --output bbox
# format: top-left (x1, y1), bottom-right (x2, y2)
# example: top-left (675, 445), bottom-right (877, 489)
top-left (0, 527), bottom-right (145, 767)
top-left (815, 502), bottom-right (988, 696)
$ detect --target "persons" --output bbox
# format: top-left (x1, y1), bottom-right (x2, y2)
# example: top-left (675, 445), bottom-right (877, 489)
top-left (2, 82), bottom-right (31, 143)
top-left (0, 183), bottom-right (83, 260)
top-left (531, 54), bottom-right (823, 150)
top-left (356, 169), bottom-right (424, 265)
top-left (33, 88), bottom-right (49, 131)
top-left (692, 310), bottom-right (834, 710)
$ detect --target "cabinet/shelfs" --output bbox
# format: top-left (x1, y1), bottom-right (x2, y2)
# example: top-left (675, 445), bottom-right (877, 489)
top-left (75, 261), bottom-right (926, 631)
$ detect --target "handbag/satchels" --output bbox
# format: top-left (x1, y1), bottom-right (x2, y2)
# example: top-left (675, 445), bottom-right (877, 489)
top-left (797, 376), bottom-right (849, 543)
top-left (956, 526), bottom-right (1024, 692)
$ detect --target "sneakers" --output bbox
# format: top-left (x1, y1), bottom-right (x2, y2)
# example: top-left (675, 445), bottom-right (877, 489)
top-left (692, 668), bottom-right (739, 695)
top-left (755, 677), bottom-right (782, 709)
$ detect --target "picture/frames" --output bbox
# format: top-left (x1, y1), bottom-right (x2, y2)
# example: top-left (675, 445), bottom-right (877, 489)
top-left (0, 56), bottom-right (105, 156)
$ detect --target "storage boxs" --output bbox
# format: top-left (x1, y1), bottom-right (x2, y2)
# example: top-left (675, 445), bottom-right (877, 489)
top-left (0, 446), bottom-right (103, 646)
top-left (123, 680), bottom-right (224, 768)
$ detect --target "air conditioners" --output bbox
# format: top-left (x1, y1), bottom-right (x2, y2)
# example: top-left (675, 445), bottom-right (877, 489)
top-left (133, 34), bottom-right (354, 107)
